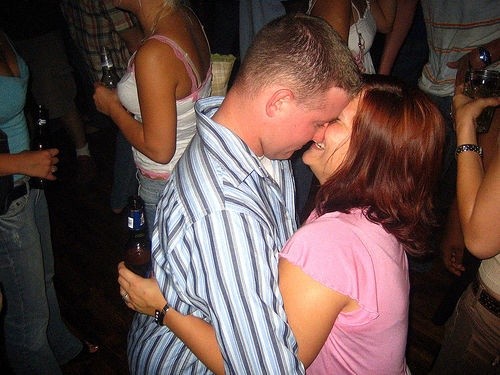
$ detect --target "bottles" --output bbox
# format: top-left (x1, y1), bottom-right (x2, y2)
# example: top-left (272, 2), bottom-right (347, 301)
top-left (29, 106), bottom-right (56, 189)
top-left (99, 45), bottom-right (121, 90)
top-left (122, 194), bottom-right (152, 278)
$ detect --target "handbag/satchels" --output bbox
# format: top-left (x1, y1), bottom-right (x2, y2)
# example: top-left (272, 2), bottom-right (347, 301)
top-left (184, 4), bottom-right (236, 97)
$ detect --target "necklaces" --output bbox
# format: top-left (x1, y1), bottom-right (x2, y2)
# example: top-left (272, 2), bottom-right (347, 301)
top-left (352, 12), bottom-right (367, 52)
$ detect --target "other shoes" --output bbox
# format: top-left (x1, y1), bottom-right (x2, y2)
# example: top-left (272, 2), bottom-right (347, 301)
top-left (76, 154), bottom-right (97, 184)
top-left (74, 342), bottom-right (98, 361)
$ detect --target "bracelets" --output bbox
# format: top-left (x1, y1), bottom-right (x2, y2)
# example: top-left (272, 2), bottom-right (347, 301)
top-left (454, 142), bottom-right (486, 158)
top-left (153, 301), bottom-right (173, 327)
top-left (476, 45), bottom-right (494, 67)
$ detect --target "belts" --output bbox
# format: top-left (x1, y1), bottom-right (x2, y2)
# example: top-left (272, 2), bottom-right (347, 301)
top-left (470, 280), bottom-right (500, 318)
top-left (12, 178), bottom-right (34, 202)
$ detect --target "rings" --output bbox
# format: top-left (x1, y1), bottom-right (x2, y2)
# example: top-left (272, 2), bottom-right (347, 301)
top-left (121, 292), bottom-right (130, 303)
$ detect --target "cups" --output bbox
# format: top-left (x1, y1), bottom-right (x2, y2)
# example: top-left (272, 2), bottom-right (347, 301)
top-left (463, 69), bottom-right (500, 135)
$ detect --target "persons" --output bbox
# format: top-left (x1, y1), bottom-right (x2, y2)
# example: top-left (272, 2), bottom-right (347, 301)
top-left (434, 82), bottom-right (500, 375)
top-left (125, 12), bottom-right (365, 375)
top-left (0, 1), bottom-right (100, 186)
top-left (379, 0), bottom-right (500, 163)
top-left (307, 0), bottom-right (397, 76)
top-left (55, 0), bottom-right (148, 216)
top-left (0, 30), bottom-right (101, 375)
top-left (117, 78), bottom-right (449, 375)
top-left (92, 1), bottom-right (212, 241)
top-left (0, 129), bottom-right (60, 216)
